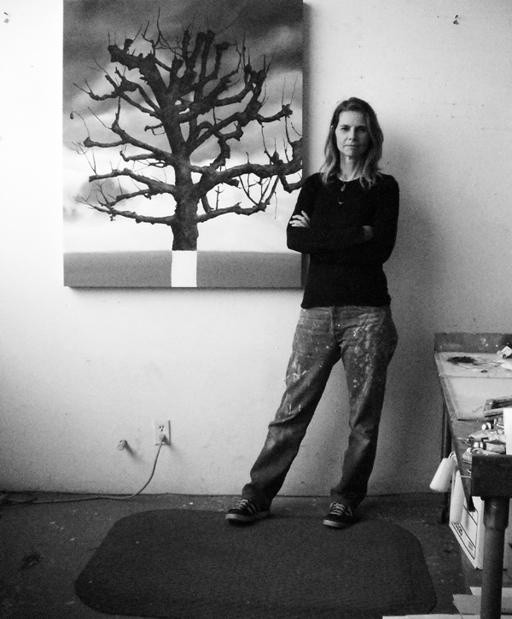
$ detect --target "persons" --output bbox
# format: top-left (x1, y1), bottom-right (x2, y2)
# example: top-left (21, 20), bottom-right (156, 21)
top-left (224, 95), bottom-right (399, 530)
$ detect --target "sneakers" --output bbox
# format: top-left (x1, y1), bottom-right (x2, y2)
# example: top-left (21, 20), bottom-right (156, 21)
top-left (222, 494), bottom-right (271, 524)
top-left (322, 497), bottom-right (359, 529)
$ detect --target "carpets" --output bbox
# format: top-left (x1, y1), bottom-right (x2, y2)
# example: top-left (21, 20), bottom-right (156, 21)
top-left (73, 507), bottom-right (438, 619)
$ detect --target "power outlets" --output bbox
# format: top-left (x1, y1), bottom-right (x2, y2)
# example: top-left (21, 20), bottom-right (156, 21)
top-left (152, 419), bottom-right (170, 448)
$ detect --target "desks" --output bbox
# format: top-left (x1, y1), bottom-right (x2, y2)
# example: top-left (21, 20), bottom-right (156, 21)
top-left (434, 328), bottom-right (512, 619)
